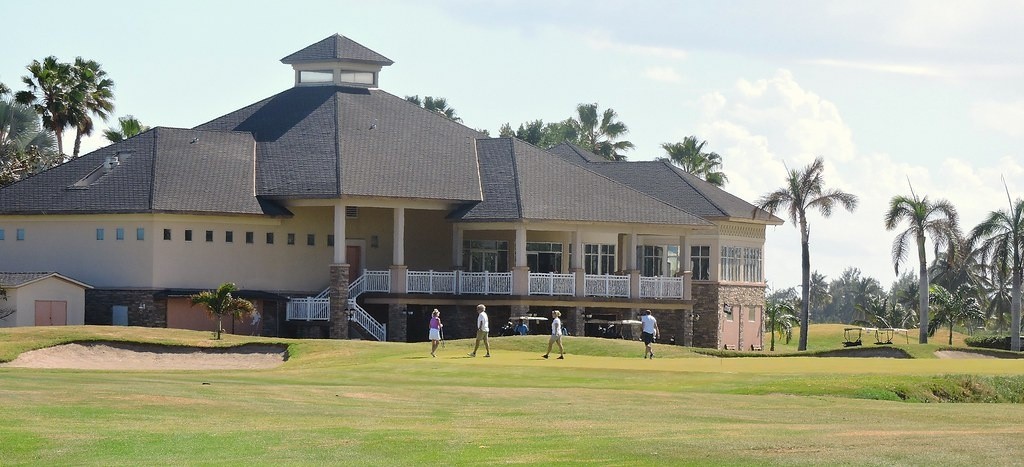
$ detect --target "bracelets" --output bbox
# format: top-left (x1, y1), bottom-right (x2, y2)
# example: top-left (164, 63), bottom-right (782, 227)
top-left (657, 335), bottom-right (660, 336)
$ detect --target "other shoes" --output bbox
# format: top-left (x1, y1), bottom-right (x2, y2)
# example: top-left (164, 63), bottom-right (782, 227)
top-left (650, 354), bottom-right (654, 359)
top-left (431, 353), bottom-right (436, 357)
top-left (483, 354), bottom-right (490, 357)
top-left (557, 356), bottom-right (564, 359)
top-left (542, 355), bottom-right (548, 358)
top-left (468, 353), bottom-right (476, 357)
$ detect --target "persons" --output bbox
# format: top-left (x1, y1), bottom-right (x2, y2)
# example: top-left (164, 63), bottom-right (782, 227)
top-left (499, 319), bottom-right (529, 337)
top-left (467, 303), bottom-right (490, 358)
top-left (639, 310), bottom-right (660, 359)
top-left (561, 323), bottom-right (568, 337)
top-left (428, 309), bottom-right (443, 358)
top-left (596, 323), bottom-right (625, 340)
top-left (541, 310), bottom-right (564, 360)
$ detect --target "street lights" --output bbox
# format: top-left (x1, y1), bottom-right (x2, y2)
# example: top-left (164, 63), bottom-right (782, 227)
top-left (345, 308), bottom-right (355, 340)
top-left (526, 311), bottom-right (538, 335)
top-left (403, 307), bottom-right (413, 343)
top-left (581, 311), bottom-right (593, 337)
top-left (689, 313), bottom-right (700, 347)
